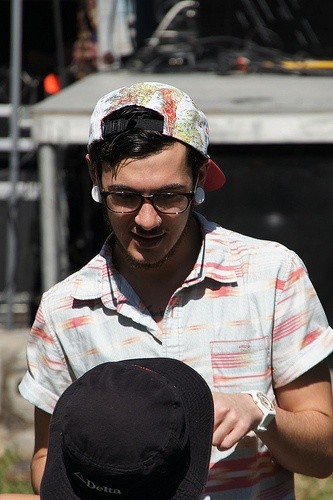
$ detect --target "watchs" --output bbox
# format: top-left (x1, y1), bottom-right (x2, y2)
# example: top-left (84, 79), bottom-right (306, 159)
top-left (243, 389), bottom-right (277, 435)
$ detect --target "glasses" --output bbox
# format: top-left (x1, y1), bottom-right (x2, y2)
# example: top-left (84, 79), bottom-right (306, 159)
top-left (97, 170), bottom-right (201, 215)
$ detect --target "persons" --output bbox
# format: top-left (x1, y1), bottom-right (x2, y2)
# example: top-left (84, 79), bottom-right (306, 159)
top-left (16, 80), bottom-right (333, 500)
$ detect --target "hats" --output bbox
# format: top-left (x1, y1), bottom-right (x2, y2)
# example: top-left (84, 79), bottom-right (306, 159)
top-left (87, 81), bottom-right (225, 192)
top-left (39, 358), bottom-right (215, 500)
